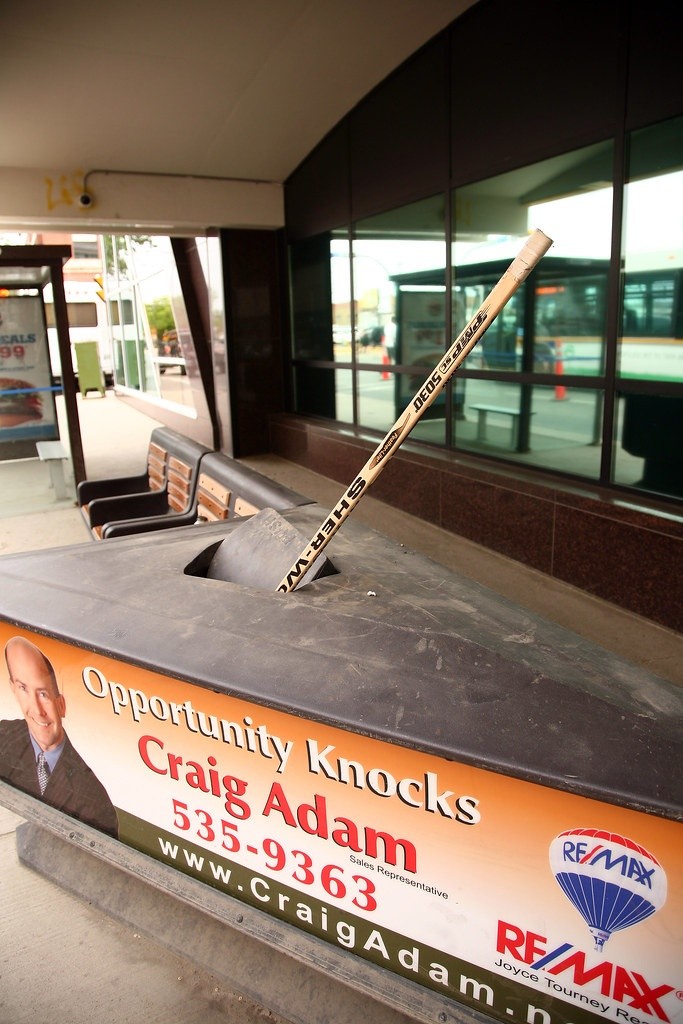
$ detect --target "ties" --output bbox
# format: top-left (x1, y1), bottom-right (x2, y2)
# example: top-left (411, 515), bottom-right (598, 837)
top-left (37, 753), bottom-right (50, 796)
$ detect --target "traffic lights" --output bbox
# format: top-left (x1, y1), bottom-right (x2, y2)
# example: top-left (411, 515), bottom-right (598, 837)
top-left (93, 274), bottom-right (106, 303)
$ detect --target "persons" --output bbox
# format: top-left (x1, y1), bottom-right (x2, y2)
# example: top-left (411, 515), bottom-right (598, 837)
top-left (0, 636), bottom-right (119, 841)
top-left (384, 316), bottom-right (397, 360)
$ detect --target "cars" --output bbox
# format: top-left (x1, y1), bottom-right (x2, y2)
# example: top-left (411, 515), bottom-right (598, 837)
top-left (333, 324), bottom-right (384, 347)
top-left (157, 329), bottom-right (226, 378)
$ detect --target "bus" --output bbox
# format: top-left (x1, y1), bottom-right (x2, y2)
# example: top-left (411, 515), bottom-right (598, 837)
top-left (380, 265), bottom-right (683, 399)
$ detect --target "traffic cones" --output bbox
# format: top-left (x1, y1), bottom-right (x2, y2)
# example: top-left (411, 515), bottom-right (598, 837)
top-left (551, 361), bottom-right (570, 403)
top-left (379, 356), bottom-right (392, 381)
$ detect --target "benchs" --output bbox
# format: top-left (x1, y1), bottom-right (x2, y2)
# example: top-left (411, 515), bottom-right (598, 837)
top-left (78, 427), bottom-right (316, 543)
top-left (468, 403), bottom-right (535, 450)
